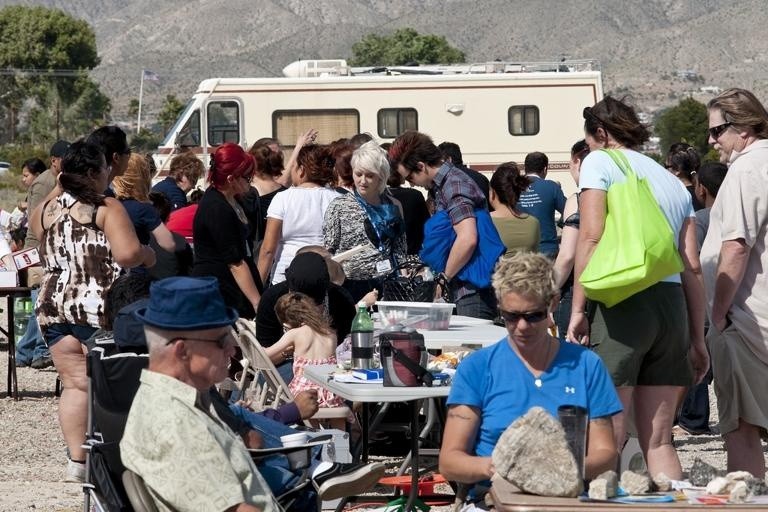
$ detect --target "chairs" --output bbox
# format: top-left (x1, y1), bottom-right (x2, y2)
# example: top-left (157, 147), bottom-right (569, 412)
top-left (231, 318), bottom-right (352, 430)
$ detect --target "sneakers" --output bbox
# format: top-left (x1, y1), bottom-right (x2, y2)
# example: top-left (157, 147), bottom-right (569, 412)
top-left (12, 351), bottom-right (54, 368)
top-left (312, 462), bottom-right (385, 500)
top-left (65, 459), bottom-right (87, 483)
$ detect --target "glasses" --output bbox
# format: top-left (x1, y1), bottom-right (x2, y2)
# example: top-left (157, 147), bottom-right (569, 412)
top-left (119, 148), bottom-right (131, 158)
top-left (239, 175), bottom-right (253, 183)
top-left (165, 333), bottom-right (231, 349)
top-left (583, 107), bottom-right (612, 132)
top-left (500, 304), bottom-right (548, 322)
top-left (405, 170), bottom-right (413, 184)
top-left (709, 122), bottom-right (733, 138)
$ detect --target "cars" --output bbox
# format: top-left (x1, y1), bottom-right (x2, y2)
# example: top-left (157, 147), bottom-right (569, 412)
top-left (0, 162), bottom-right (11, 170)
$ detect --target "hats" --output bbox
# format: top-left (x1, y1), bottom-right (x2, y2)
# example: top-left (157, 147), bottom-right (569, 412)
top-left (50, 140), bottom-right (71, 158)
top-left (287, 253), bottom-right (330, 314)
top-left (113, 299), bottom-right (150, 345)
top-left (294, 246), bottom-right (345, 285)
top-left (135, 277), bottom-right (239, 329)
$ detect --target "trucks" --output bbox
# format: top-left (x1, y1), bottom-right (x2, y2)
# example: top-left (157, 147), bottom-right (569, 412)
top-left (150, 58), bottom-right (608, 220)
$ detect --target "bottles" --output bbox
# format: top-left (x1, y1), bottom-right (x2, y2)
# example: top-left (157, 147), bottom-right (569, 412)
top-left (14, 296), bottom-right (33, 351)
top-left (350, 301), bottom-right (373, 334)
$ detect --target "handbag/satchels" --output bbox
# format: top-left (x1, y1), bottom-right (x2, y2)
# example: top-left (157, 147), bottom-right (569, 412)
top-left (421, 207), bottom-right (507, 287)
top-left (380, 331), bottom-right (426, 387)
top-left (383, 261), bottom-right (437, 301)
top-left (579, 173), bottom-right (685, 309)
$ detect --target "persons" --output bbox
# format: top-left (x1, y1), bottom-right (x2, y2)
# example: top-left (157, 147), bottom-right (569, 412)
top-left (0, 88), bottom-right (768, 510)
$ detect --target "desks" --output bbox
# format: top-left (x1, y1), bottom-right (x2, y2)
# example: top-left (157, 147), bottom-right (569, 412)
top-left (0, 287), bottom-right (31, 400)
top-left (337, 314), bottom-right (509, 347)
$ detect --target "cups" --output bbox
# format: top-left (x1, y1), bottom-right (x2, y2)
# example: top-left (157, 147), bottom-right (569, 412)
top-left (557, 402), bottom-right (589, 484)
top-left (351, 329), bottom-right (375, 370)
